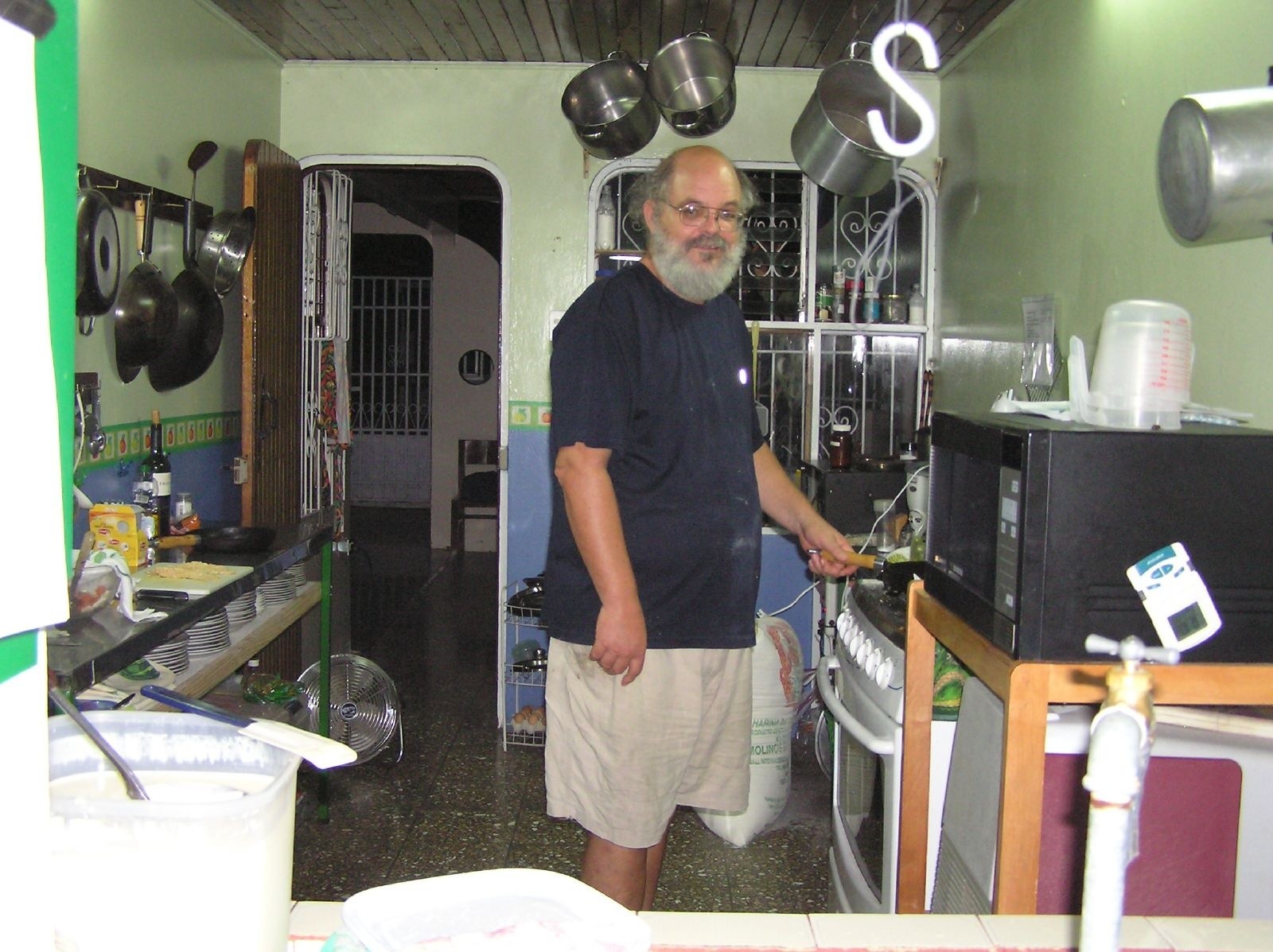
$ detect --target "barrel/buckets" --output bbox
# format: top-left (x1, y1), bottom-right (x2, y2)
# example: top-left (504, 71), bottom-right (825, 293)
top-left (789, 42), bottom-right (921, 198)
top-left (647, 30), bottom-right (739, 139)
top-left (560, 51), bottom-right (661, 160)
top-left (48, 712), bottom-right (306, 952)
top-left (1156, 84), bottom-right (1273, 246)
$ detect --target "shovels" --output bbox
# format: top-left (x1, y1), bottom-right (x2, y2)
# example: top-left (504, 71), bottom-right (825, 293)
top-left (141, 685), bottom-right (359, 770)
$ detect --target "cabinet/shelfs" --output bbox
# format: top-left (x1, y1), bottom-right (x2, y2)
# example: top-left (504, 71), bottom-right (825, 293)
top-left (500, 578), bottom-right (551, 752)
top-left (44, 522), bottom-right (339, 783)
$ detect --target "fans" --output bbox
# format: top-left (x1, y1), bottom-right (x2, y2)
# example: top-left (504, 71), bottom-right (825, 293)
top-left (294, 652), bottom-right (404, 769)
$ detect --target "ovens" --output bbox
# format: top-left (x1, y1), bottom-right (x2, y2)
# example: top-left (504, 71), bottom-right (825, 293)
top-left (813, 655), bottom-right (903, 914)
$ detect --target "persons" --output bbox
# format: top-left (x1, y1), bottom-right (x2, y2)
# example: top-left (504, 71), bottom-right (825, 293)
top-left (542, 145), bottom-right (863, 914)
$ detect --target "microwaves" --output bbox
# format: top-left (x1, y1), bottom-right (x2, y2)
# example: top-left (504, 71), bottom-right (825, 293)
top-left (923, 410), bottom-right (1273, 668)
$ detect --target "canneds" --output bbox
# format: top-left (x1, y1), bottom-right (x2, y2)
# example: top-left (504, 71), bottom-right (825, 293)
top-left (830, 424), bottom-right (854, 467)
top-left (880, 294), bottom-right (907, 324)
top-left (863, 292), bottom-right (880, 324)
top-left (815, 279), bottom-right (863, 324)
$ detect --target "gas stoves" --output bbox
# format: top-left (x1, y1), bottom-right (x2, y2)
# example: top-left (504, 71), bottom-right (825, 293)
top-left (835, 575), bottom-right (907, 726)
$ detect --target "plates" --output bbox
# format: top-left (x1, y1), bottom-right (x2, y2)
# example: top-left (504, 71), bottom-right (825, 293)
top-left (341, 868), bottom-right (652, 952)
top-left (143, 562), bottom-right (306, 676)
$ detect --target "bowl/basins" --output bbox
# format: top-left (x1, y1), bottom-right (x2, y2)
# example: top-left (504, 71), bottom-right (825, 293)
top-left (54, 565), bottom-right (120, 633)
top-left (204, 527), bottom-right (276, 554)
top-left (83, 700), bottom-right (117, 711)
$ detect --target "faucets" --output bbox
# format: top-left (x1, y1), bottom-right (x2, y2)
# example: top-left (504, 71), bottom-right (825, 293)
top-left (1078, 634), bottom-right (1177, 820)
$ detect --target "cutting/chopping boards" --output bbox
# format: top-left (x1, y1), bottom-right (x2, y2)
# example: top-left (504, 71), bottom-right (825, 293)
top-left (131, 562), bottom-right (253, 595)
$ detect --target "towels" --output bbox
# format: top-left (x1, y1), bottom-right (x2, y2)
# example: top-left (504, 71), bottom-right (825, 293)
top-left (80, 549), bottom-right (169, 624)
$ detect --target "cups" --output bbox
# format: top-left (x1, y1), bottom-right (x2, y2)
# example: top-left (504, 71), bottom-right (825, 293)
top-left (1084, 300), bottom-right (1197, 431)
top-left (814, 283), bottom-right (833, 321)
top-left (873, 498), bottom-right (897, 553)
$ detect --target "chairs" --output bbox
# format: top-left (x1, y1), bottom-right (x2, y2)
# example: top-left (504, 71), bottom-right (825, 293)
top-left (448, 438), bottom-right (500, 566)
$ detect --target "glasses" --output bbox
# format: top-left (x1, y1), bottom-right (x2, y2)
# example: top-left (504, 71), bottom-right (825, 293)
top-left (654, 195), bottom-right (749, 232)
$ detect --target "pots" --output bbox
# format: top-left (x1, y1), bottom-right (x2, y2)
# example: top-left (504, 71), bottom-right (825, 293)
top-left (78, 165), bottom-right (226, 392)
top-left (807, 547), bottom-right (927, 589)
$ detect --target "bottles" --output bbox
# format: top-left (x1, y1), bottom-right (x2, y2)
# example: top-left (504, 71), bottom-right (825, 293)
top-left (830, 424), bottom-right (852, 471)
top-left (132, 465), bottom-right (161, 541)
top-left (907, 284), bottom-right (926, 326)
top-left (172, 492), bottom-right (194, 519)
top-left (881, 293), bottom-right (907, 324)
top-left (863, 292), bottom-right (880, 324)
top-left (142, 410), bottom-right (172, 537)
top-left (844, 280), bottom-right (865, 323)
